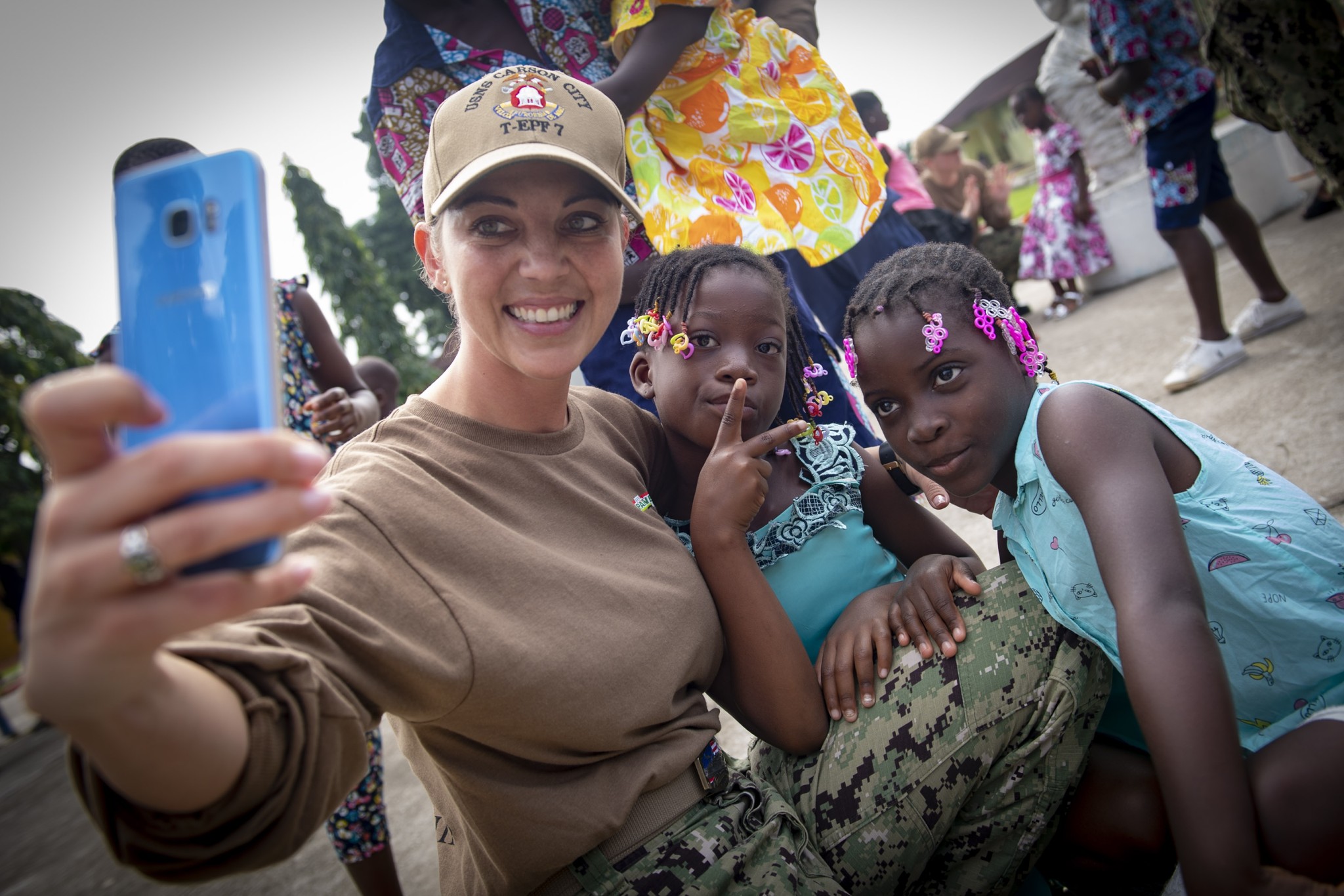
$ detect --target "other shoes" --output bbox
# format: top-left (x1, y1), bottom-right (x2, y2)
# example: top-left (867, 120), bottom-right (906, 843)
top-left (1303, 194), bottom-right (1340, 220)
top-left (1045, 289), bottom-right (1085, 317)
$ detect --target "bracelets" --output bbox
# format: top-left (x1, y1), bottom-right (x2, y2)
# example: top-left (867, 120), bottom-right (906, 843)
top-left (879, 441), bottom-right (919, 496)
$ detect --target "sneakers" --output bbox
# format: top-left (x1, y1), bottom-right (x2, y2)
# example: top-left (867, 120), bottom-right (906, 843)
top-left (1162, 331), bottom-right (1249, 395)
top-left (1229, 292), bottom-right (1305, 344)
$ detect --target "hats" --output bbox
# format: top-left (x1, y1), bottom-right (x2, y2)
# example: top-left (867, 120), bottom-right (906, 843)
top-left (417, 64), bottom-right (648, 226)
top-left (916, 123), bottom-right (969, 157)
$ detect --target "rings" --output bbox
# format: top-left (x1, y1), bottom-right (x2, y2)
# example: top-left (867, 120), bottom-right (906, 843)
top-left (121, 525), bottom-right (163, 586)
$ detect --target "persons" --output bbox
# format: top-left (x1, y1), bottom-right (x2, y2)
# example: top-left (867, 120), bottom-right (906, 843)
top-left (844, 244), bottom-right (1344, 896)
top-left (366, 0), bottom-right (1020, 548)
top-left (630, 245), bottom-right (986, 896)
top-left (21, 67), bottom-right (1113, 896)
top-left (1079, 0), bottom-right (1309, 392)
top-left (90, 138), bottom-right (404, 896)
top-left (851, 86), bottom-right (1110, 315)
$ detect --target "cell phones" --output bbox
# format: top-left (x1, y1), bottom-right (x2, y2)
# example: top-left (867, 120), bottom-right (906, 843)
top-left (108, 149), bottom-right (286, 575)
